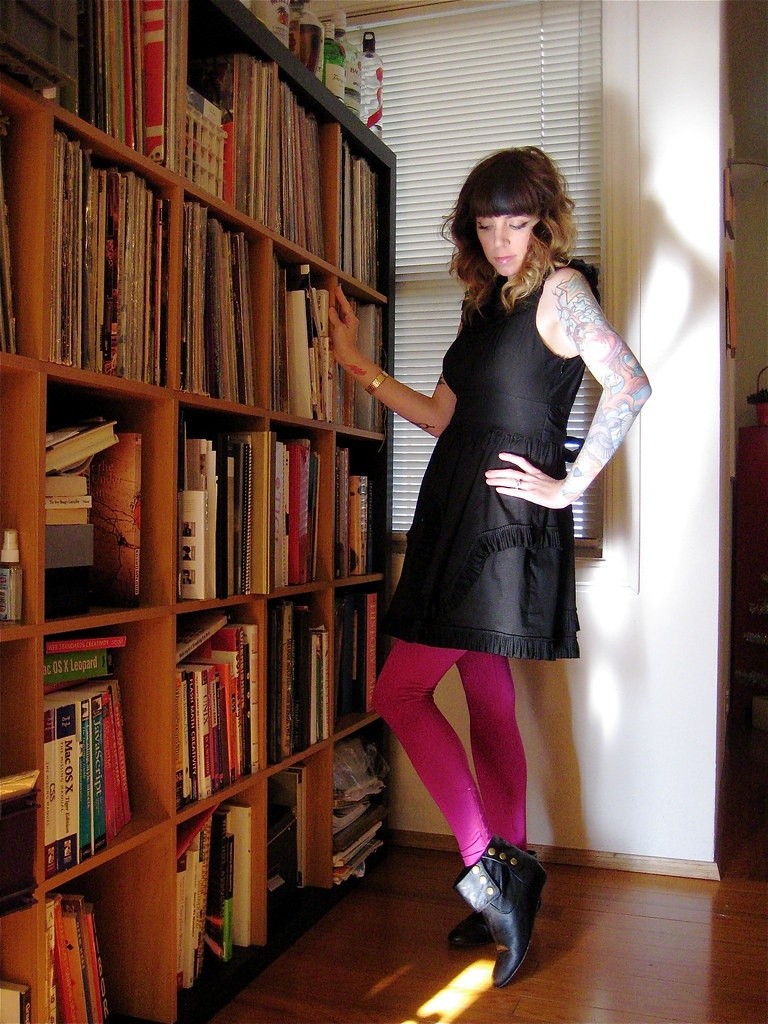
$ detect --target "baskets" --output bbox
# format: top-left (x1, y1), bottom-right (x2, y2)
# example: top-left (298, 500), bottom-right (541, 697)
top-left (757, 367), bottom-right (768, 426)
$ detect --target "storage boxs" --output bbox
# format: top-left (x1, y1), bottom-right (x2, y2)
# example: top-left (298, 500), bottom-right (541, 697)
top-left (45, 524), bottom-right (95, 569)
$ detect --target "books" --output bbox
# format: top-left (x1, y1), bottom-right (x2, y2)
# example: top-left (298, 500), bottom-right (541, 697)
top-left (0, 0), bottom-right (385, 1024)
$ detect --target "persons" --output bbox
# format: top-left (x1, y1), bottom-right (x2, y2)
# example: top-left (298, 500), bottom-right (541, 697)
top-left (326, 147), bottom-right (654, 986)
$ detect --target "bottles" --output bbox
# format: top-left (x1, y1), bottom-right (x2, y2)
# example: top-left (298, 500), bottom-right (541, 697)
top-left (241, 0), bottom-right (383, 141)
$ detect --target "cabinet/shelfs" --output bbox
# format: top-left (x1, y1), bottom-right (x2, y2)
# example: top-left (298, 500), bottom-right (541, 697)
top-left (0, 0), bottom-right (395, 1024)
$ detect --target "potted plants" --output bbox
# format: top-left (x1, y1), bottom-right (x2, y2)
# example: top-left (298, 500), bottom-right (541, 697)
top-left (746, 388), bottom-right (768, 426)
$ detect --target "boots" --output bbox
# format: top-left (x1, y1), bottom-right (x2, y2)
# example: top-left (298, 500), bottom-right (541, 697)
top-left (448, 850), bottom-right (541, 947)
top-left (453, 834), bottom-right (547, 987)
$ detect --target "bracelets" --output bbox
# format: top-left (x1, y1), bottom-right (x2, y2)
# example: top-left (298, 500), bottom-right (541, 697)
top-left (364, 370), bottom-right (389, 396)
